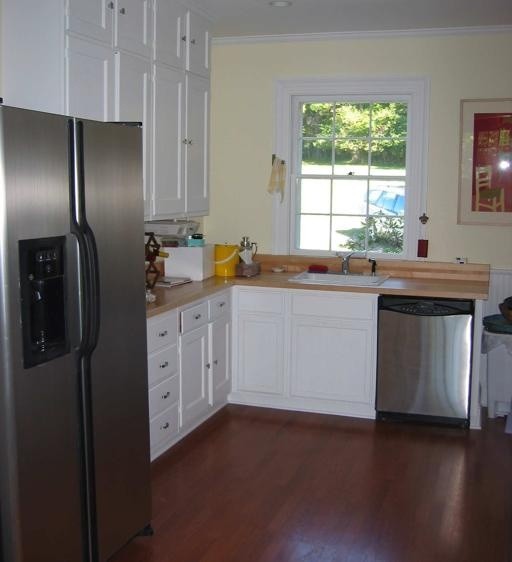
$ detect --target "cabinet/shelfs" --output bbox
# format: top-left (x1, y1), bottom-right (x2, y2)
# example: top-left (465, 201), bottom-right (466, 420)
top-left (66, 34), bottom-right (144, 126)
top-left (178, 289), bottom-right (230, 438)
top-left (231, 287), bottom-right (486, 431)
top-left (0, 0), bottom-right (67, 116)
top-left (145, 0), bottom-right (213, 79)
top-left (66, 0), bottom-right (150, 56)
top-left (146, 306), bottom-right (179, 453)
top-left (145, 62), bottom-right (208, 221)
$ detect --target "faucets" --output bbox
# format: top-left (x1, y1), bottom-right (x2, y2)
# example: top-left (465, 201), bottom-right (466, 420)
top-left (342, 251), bottom-right (356, 275)
top-left (369, 258), bottom-right (377, 277)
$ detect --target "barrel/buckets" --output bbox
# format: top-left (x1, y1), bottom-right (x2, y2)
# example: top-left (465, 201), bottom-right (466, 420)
top-left (214, 244), bottom-right (239, 277)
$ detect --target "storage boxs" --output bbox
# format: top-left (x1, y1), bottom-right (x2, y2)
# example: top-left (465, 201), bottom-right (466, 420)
top-left (159, 243), bottom-right (216, 282)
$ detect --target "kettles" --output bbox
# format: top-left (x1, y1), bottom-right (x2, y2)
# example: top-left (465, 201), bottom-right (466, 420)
top-left (238, 237), bottom-right (257, 263)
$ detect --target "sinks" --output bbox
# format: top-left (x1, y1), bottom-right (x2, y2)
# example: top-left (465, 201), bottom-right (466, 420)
top-left (286, 268), bottom-right (392, 289)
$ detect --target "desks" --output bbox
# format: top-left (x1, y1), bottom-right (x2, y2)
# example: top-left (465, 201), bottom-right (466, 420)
top-left (479, 313), bottom-right (512, 434)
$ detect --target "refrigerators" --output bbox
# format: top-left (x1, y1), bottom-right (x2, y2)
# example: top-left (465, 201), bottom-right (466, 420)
top-left (0, 104), bottom-right (153, 561)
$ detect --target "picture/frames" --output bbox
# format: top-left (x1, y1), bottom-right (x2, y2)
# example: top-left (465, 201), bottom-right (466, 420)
top-left (456, 97), bottom-right (512, 228)
